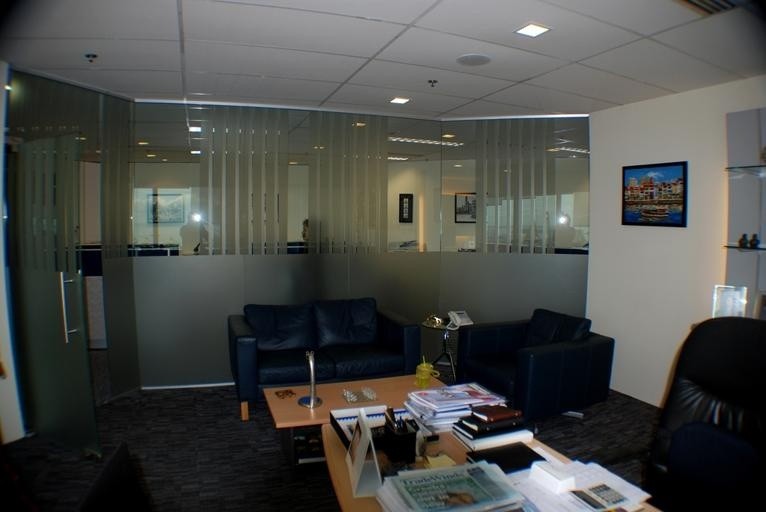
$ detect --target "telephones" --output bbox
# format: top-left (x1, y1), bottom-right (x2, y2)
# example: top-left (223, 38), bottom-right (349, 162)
top-left (448, 310), bottom-right (474, 327)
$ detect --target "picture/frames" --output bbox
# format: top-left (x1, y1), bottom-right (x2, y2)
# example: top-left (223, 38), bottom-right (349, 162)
top-left (712, 285), bottom-right (748, 319)
top-left (621, 161), bottom-right (687, 227)
top-left (455, 192), bottom-right (478, 223)
top-left (147, 193), bottom-right (185, 223)
top-left (400, 193), bottom-right (412, 223)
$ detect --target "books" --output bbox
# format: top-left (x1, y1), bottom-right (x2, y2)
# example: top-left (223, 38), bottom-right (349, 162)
top-left (403, 380), bottom-right (549, 478)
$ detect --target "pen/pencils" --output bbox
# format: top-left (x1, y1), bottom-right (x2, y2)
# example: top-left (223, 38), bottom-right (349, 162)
top-left (384, 409), bottom-right (407, 432)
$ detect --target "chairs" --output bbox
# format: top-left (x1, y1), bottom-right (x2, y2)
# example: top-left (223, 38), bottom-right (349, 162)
top-left (458, 309), bottom-right (614, 436)
top-left (643, 317), bottom-right (765, 511)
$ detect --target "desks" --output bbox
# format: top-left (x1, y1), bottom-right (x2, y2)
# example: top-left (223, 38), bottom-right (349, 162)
top-left (424, 315), bottom-right (471, 382)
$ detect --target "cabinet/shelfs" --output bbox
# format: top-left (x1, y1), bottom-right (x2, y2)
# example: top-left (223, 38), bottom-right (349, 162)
top-left (725, 107), bottom-right (765, 320)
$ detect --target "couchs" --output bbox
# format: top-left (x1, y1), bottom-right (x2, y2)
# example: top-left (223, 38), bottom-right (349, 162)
top-left (227, 297), bottom-right (422, 421)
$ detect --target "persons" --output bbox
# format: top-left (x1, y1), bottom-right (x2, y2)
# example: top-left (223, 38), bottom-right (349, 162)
top-left (177, 209), bottom-right (210, 257)
top-left (301, 218), bottom-right (334, 253)
